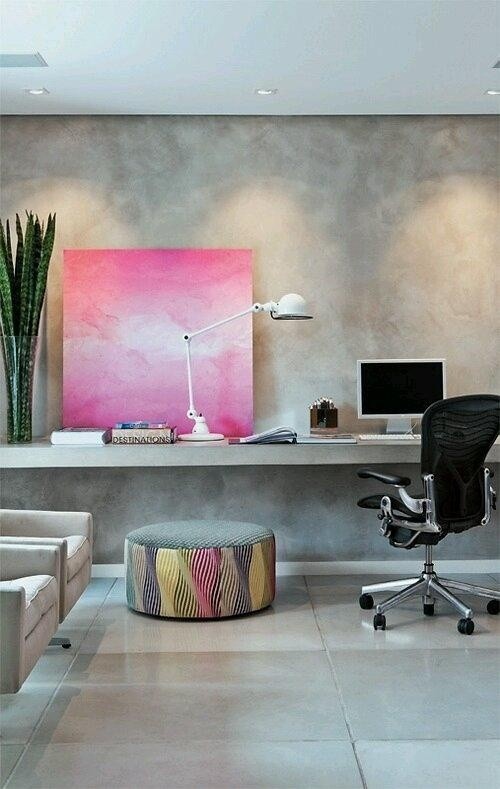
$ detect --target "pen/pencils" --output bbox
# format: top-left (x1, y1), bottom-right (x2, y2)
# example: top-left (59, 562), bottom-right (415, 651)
top-left (310, 396), bottom-right (336, 410)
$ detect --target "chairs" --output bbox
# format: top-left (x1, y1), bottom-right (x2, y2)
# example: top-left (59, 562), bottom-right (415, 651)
top-left (0, 508), bottom-right (94, 694)
top-left (356, 392), bottom-right (499, 635)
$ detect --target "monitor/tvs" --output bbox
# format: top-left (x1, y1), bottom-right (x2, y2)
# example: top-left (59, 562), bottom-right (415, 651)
top-left (356, 358), bottom-right (447, 434)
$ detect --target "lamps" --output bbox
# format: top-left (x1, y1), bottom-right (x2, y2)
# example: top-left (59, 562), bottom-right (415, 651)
top-left (177, 292), bottom-right (314, 442)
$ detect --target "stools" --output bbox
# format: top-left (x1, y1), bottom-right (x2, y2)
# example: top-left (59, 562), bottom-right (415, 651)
top-left (123, 518), bottom-right (276, 623)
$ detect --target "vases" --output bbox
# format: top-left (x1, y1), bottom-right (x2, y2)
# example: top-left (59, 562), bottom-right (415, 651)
top-left (0, 333), bottom-right (40, 445)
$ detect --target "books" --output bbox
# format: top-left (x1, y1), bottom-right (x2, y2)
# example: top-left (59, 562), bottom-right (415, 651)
top-left (228, 426), bottom-right (357, 445)
top-left (50, 419), bottom-right (175, 445)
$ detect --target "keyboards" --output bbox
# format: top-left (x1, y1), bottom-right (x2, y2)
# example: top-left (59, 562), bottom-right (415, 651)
top-left (359, 435), bottom-right (421, 441)
